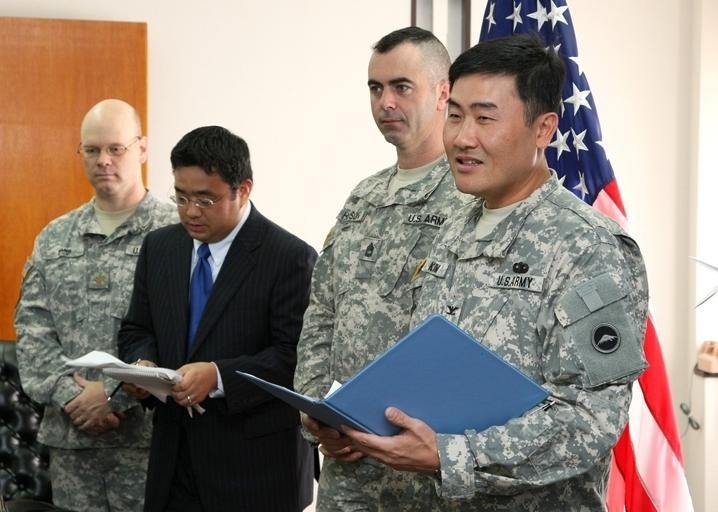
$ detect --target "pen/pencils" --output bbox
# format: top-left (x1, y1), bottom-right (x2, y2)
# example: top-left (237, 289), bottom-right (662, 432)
top-left (106, 357), bottom-right (141, 401)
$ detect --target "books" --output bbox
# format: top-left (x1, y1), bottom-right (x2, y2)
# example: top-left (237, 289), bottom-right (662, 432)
top-left (234, 311), bottom-right (550, 445)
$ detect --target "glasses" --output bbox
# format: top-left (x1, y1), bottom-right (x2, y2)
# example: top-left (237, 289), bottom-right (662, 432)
top-left (76, 140), bottom-right (138, 157)
top-left (169, 188), bottom-right (232, 209)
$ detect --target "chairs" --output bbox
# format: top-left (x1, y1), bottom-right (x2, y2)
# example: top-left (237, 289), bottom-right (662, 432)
top-left (0, 340), bottom-right (78, 512)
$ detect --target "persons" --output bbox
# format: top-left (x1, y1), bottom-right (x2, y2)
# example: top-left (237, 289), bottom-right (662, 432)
top-left (116, 123), bottom-right (321, 511)
top-left (299, 29), bottom-right (651, 510)
top-left (290, 26), bottom-right (477, 510)
top-left (9, 98), bottom-right (184, 512)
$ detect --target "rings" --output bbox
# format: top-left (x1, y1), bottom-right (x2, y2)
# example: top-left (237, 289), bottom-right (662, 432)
top-left (78, 414), bottom-right (86, 422)
top-left (187, 395), bottom-right (193, 407)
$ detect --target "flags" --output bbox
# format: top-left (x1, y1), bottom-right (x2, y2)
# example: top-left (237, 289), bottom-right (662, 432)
top-left (477, 0), bottom-right (695, 511)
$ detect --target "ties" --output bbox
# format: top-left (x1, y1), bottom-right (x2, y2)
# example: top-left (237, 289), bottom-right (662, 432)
top-left (187, 243), bottom-right (213, 353)
